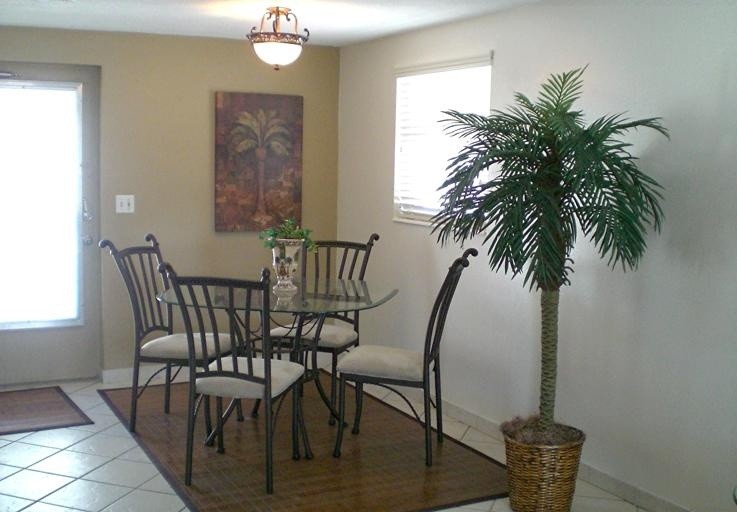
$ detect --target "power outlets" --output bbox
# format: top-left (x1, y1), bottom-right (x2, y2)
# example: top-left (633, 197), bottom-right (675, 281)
top-left (115, 194), bottom-right (136, 215)
top-left (114, 194), bottom-right (134, 215)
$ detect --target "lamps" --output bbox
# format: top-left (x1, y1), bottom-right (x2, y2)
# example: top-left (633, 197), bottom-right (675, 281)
top-left (246, 6), bottom-right (310, 71)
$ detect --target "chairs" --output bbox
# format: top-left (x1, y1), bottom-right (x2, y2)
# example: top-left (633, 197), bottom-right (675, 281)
top-left (98, 231), bottom-right (248, 446)
top-left (155, 260), bottom-right (307, 495)
top-left (332, 248), bottom-right (477, 468)
top-left (267, 232), bottom-right (380, 427)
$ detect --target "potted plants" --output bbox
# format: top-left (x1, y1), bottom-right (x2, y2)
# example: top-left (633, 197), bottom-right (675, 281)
top-left (426, 63), bottom-right (672, 512)
top-left (257, 216), bottom-right (318, 301)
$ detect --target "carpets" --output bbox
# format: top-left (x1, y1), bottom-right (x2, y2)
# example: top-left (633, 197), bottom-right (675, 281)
top-left (97, 367), bottom-right (509, 511)
top-left (0, 385), bottom-right (95, 435)
